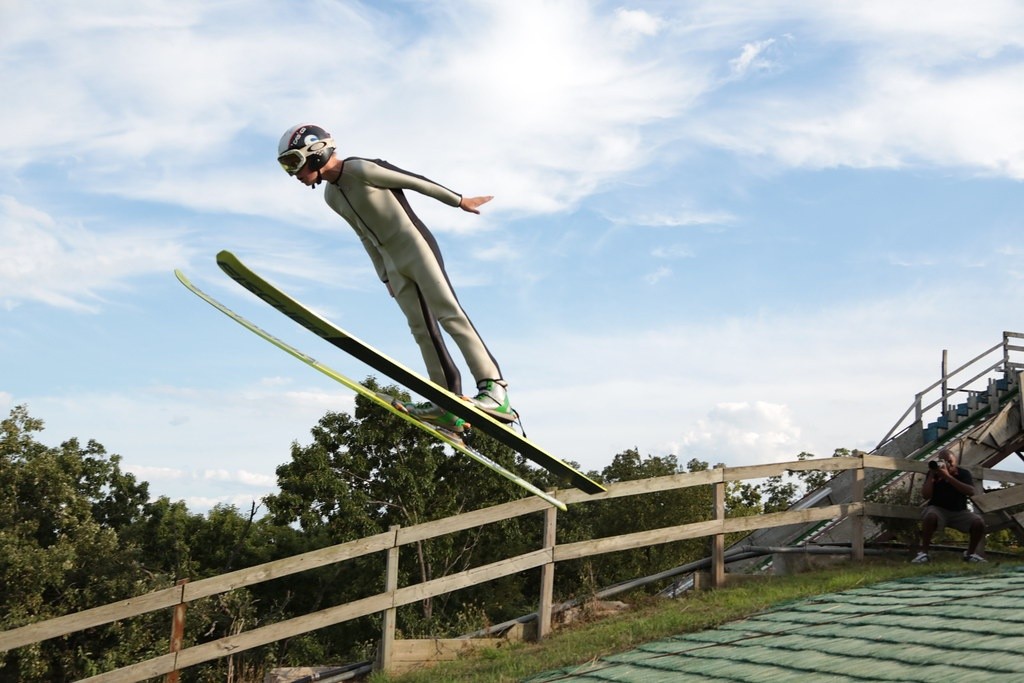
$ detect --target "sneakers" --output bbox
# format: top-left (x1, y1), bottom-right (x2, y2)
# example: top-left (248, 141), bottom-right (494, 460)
top-left (461, 378), bottom-right (516, 424)
top-left (402, 401), bottom-right (470, 433)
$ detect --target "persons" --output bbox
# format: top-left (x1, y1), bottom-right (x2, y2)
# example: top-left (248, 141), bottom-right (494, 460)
top-left (911, 450), bottom-right (987, 563)
top-left (277, 123), bottom-right (515, 432)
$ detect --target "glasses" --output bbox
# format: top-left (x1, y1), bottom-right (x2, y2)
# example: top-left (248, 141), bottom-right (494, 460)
top-left (278, 149), bottom-right (306, 176)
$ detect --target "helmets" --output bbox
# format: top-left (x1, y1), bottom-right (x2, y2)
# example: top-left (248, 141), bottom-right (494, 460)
top-left (276, 123), bottom-right (335, 172)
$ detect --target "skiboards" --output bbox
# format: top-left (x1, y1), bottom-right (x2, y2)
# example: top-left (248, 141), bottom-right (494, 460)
top-left (172, 247), bottom-right (608, 510)
